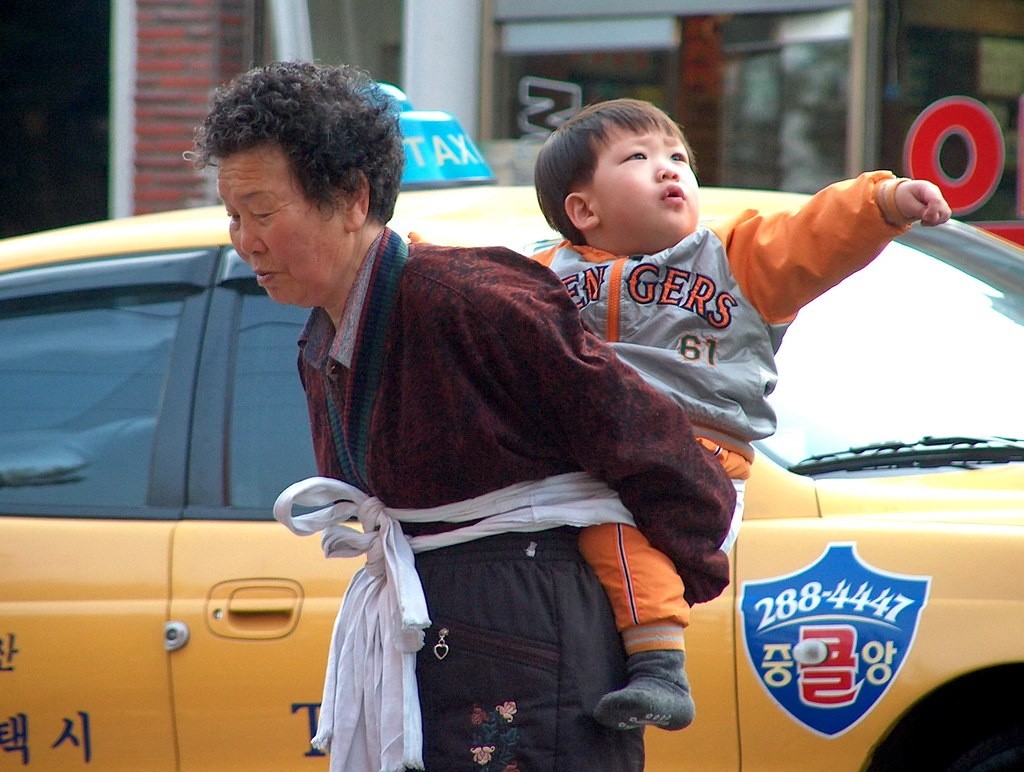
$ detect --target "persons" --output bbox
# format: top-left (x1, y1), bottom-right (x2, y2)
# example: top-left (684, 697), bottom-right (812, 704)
top-left (406, 98), bottom-right (952, 731)
top-left (193, 58), bottom-right (737, 770)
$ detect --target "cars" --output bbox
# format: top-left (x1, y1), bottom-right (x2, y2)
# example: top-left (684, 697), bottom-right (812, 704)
top-left (1, 83), bottom-right (1024, 772)
